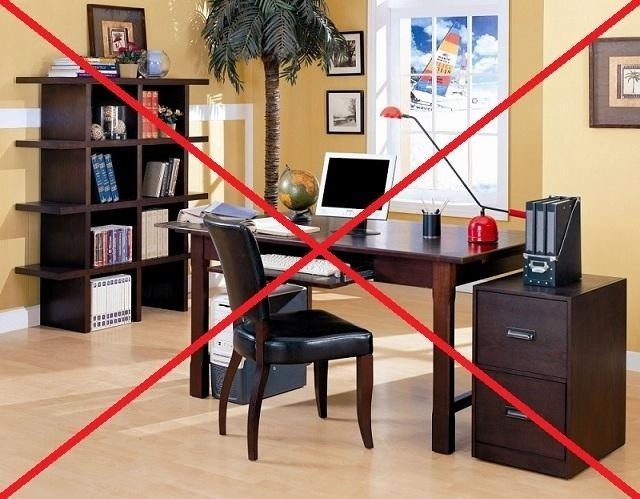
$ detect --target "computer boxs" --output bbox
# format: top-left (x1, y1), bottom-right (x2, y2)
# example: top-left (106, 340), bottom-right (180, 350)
top-left (207, 283), bottom-right (306, 405)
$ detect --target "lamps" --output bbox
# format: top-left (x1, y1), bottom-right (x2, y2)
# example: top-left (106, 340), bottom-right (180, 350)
top-left (381, 105), bottom-right (527, 245)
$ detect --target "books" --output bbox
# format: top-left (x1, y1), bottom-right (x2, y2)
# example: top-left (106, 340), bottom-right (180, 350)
top-left (90, 224), bottom-right (133, 268)
top-left (142, 90), bottom-right (159, 139)
top-left (91, 154), bottom-right (119, 204)
top-left (142, 158), bottom-right (180, 197)
top-left (142, 208), bottom-right (169, 260)
top-left (48, 58), bottom-right (118, 78)
top-left (177, 200), bottom-right (320, 237)
top-left (90, 273), bottom-right (131, 332)
top-left (526, 198), bottom-right (573, 257)
top-left (101, 106), bottom-right (126, 141)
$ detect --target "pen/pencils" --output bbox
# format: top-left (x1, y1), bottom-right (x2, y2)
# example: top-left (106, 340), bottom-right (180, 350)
top-left (420, 198), bottom-right (450, 214)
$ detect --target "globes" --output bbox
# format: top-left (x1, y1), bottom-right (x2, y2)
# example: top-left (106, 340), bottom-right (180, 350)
top-left (279, 168), bottom-right (319, 222)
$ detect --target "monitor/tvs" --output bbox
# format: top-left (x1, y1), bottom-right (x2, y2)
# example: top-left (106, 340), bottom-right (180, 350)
top-left (314, 151), bottom-right (398, 236)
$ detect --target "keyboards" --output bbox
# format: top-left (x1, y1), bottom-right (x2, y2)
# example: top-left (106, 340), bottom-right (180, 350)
top-left (261, 253), bottom-right (352, 277)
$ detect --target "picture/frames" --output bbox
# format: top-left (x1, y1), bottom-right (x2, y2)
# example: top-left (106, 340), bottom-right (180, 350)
top-left (326, 30), bottom-right (364, 133)
top-left (85, 5), bottom-right (147, 75)
top-left (587, 38), bottom-right (639, 129)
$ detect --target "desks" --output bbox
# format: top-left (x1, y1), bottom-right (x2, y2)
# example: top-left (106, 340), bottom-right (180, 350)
top-left (152, 211), bottom-right (526, 454)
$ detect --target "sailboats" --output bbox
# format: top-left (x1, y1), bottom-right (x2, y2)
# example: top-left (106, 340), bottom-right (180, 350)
top-left (410, 22), bottom-right (468, 112)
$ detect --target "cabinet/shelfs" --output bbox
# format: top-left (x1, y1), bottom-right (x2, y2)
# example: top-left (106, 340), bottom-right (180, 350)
top-left (471, 272), bottom-right (625, 480)
top-left (15, 75), bottom-right (211, 335)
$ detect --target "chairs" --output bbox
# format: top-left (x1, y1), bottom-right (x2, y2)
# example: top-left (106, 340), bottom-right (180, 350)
top-left (205, 217), bottom-right (374, 459)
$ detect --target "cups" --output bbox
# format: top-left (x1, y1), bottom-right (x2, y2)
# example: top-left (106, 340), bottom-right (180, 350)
top-left (423, 212), bottom-right (441, 240)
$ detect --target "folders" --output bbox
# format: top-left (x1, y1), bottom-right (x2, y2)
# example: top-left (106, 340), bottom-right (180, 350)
top-left (523, 195), bottom-right (580, 286)
top-left (177, 202), bottom-right (257, 224)
